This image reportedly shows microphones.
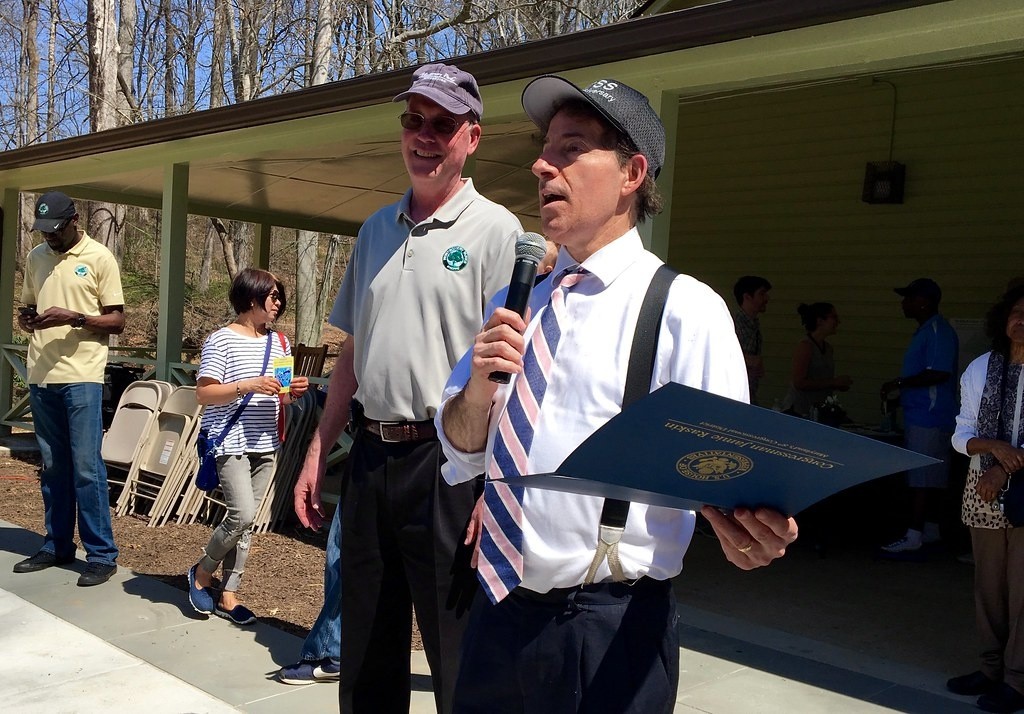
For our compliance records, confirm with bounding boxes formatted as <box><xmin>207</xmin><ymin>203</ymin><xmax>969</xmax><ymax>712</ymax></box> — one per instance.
<box><xmin>488</xmin><ymin>232</ymin><xmax>547</xmax><ymax>384</ymax></box>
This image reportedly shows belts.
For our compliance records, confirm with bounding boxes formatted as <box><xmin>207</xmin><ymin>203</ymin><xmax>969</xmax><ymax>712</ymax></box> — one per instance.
<box><xmin>511</xmin><ymin>584</ymin><xmax>646</xmax><ymax>605</ymax></box>
<box><xmin>364</xmin><ymin>417</ymin><xmax>419</xmax><ymax>442</ymax></box>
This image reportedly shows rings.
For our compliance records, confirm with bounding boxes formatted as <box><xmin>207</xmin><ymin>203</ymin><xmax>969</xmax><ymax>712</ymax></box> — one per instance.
<box><xmin>738</xmin><ymin>535</ymin><xmax>754</xmax><ymax>552</ymax></box>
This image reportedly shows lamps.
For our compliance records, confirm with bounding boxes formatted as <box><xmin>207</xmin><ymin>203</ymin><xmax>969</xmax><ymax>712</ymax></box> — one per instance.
<box><xmin>862</xmin><ymin>159</ymin><xmax>906</xmax><ymax>205</ymax></box>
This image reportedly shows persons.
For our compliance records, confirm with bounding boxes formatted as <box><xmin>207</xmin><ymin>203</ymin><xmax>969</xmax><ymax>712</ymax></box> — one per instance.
<box><xmin>781</xmin><ymin>302</ymin><xmax>853</xmax><ymax>415</ymax></box>
<box><xmin>296</xmin><ymin>63</ymin><xmax>524</xmax><ymax>714</ymax></box>
<box><xmin>880</xmin><ymin>278</ymin><xmax>959</xmax><ymax>568</ymax></box>
<box><xmin>13</xmin><ymin>189</ymin><xmax>126</xmax><ymax>584</ymax></box>
<box><xmin>946</xmin><ymin>284</ymin><xmax>1024</xmax><ymax>714</ymax></box>
<box><xmin>434</xmin><ymin>74</ymin><xmax>798</xmax><ymax>714</ymax></box>
<box><xmin>537</xmin><ymin>241</ymin><xmax>558</xmax><ymax>274</ymax></box>
<box><xmin>188</xmin><ymin>267</ymin><xmax>309</xmax><ymax>625</ymax></box>
<box><xmin>278</xmin><ymin>503</ymin><xmax>342</xmax><ymax>683</ymax></box>
<box><xmin>696</xmin><ymin>275</ymin><xmax>772</xmax><ymax>538</ymax></box>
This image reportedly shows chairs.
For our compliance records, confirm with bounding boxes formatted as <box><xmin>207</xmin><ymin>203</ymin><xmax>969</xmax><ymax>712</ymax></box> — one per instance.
<box><xmin>100</xmin><ymin>341</ymin><xmax>328</xmax><ymax>534</ymax></box>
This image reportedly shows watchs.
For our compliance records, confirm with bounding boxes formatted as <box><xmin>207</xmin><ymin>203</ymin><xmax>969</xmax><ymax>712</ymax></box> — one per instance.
<box><xmin>896</xmin><ymin>377</ymin><xmax>903</xmax><ymax>388</ymax></box>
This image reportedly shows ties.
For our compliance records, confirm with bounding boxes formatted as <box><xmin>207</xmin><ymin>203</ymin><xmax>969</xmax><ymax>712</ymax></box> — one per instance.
<box><xmin>477</xmin><ymin>268</ymin><xmax>591</xmax><ymax>605</ymax></box>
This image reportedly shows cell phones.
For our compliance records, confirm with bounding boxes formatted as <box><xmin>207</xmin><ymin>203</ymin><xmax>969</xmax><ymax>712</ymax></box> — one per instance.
<box><xmin>18</xmin><ymin>307</ymin><xmax>38</xmax><ymax>318</ymax></box>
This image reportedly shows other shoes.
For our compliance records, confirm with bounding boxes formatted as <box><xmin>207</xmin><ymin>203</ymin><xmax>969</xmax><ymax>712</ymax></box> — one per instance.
<box><xmin>187</xmin><ymin>563</ymin><xmax>213</xmax><ymax>614</ymax></box>
<box><xmin>976</xmin><ymin>680</ymin><xmax>1024</xmax><ymax>712</ymax></box>
<box><xmin>215</xmin><ymin>596</ymin><xmax>257</xmax><ymax>624</ymax></box>
<box><xmin>881</xmin><ymin>529</ymin><xmax>921</xmax><ymax>552</ymax></box>
<box><xmin>945</xmin><ymin>671</ymin><xmax>992</xmax><ymax>695</ymax></box>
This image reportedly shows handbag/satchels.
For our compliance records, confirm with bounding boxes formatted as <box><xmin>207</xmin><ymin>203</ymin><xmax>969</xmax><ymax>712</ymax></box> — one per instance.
<box><xmin>194</xmin><ymin>428</ymin><xmax>220</xmax><ymax>491</ymax></box>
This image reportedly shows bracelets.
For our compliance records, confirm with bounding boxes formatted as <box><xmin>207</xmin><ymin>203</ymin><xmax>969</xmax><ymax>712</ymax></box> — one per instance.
<box><xmin>237</xmin><ymin>384</ymin><xmax>241</xmax><ymax>397</ymax></box>
<box><xmin>78</xmin><ymin>313</ymin><xmax>85</xmax><ymax>327</ymax></box>
<box><xmin>289</xmin><ymin>391</ymin><xmax>303</xmax><ymax>401</ymax></box>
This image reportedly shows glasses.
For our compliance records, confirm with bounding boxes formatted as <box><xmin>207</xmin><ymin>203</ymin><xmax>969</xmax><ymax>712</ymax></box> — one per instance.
<box><xmin>268</xmin><ymin>290</ymin><xmax>284</xmax><ymax>302</ymax></box>
<box><xmin>398</xmin><ymin>111</ymin><xmax>469</xmax><ymax>134</ymax></box>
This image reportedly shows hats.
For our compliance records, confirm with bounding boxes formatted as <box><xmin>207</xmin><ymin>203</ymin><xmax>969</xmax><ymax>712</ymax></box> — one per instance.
<box><xmin>29</xmin><ymin>190</ymin><xmax>75</xmax><ymax>233</ymax></box>
<box><xmin>521</xmin><ymin>74</ymin><xmax>665</xmax><ymax>182</ymax></box>
<box><xmin>894</xmin><ymin>278</ymin><xmax>942</xmax><ymax>305</ymax></box>
<box><xmin>392</xmin><ymin>63</ymin><xmax>483</xmax><ymax>122</ymax></box>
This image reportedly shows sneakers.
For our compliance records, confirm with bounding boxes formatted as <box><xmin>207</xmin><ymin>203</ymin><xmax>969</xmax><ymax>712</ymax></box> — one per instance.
<box><xmin>277</xmin><ymin>657</ymin><xmax>340</xmax><ymax>684</ymax></box>
<box><xmin>77</xmin><ymin>562</ymin><xmax>117</xmax><ymax>585</ymax></box>
<box><xmin>14</xmin><ymin>551</ymin><xmax>71</xmax><ymax>572</ymax></box>
